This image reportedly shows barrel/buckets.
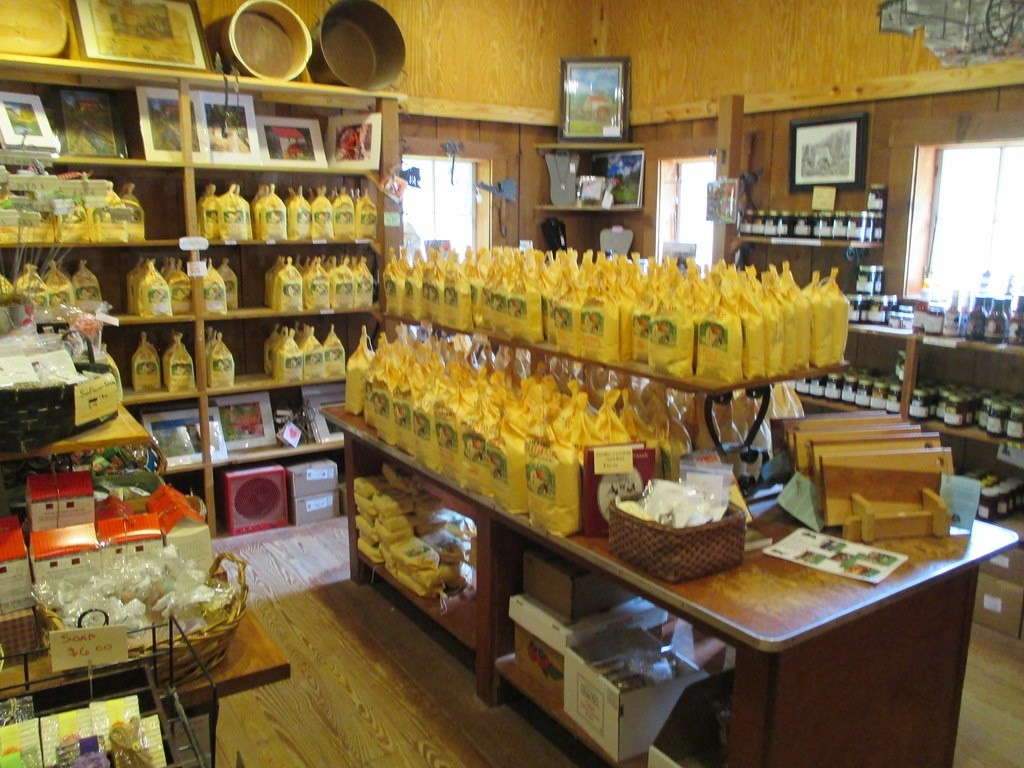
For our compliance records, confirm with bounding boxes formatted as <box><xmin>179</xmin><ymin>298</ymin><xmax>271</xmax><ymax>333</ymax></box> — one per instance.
<box><xmin>307</xmin><ymin>0</ymin><xmax>405</xmax><ymax>93</ymax></box>
<box><xmin>219</xmin><ymin>0</ymin><xmax>312</xmax><ymax>83</ymax></box>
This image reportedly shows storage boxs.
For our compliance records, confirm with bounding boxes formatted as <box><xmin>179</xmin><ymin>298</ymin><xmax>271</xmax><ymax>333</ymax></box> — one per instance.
<box><xmin>509</xmin><ymin>546</ymin><xmax>735</xmax><ymax>768</ymax></box>
<box><xmin>225</xmin><ymin>460</ymin><xmax>347</xmax><ymax>536</ymax></box>
<box><xmin>971</xmin><ymin>549</ymin><xmax>1024</xmax><ymax>639</ymax></box>
<box><xmin>0</xmin><ymin>471</ymin><xmax>211</xmax><ymax>657</ymax></box>
<box><xmin>0</xmin><ymin>149</ymin><xmax>107</xmax><ymax>226</ymax></box>
<box><xmin>0</xmin><ymin>695</ymin><xmax>168</xmax><ymax>768</ymax></box>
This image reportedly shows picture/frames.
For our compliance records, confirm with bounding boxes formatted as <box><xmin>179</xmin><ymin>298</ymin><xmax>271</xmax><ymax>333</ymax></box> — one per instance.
<box><xmin>0</xmin><ymin>85</ymin><xmax>383</xmax><ymax>171</ymax></box>
<box><xmin>788</xmin><ymin>110</ymin><xmax>869</xmax><ymax>192</ymax></box>
<box><xmin>558</xmin><ymin>55</ymin><xmax>631</xmax><ymax>144</ymax></box>
<box><xmin>140</xmin><ymin>383</ymin><xmax>345</xmax><ymax>468</ymax></box>
<box><xmin>70</xmin><ymin>0</ymin><xmax>215</xmax><ymax>72</ymax></box>
<box><xmin>591</xmin><ymin>150</ymin><xmax>645</xmax><ymax>209</ymax></box>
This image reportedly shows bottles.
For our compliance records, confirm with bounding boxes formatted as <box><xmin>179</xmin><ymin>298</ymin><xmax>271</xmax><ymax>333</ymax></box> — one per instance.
<box><xmin>941</xmin><ymin>290</ymin><xmax>1024</xmax><ymax>347</ymax></box>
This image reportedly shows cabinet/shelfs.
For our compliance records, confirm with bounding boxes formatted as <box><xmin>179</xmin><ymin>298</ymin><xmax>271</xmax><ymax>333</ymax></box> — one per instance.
<box><xmin>532</xmin><ymin>143</ymin><xmax>645</xmax><ymax>210</ymax></box>
<box><xmin>793</xmin><ymin>322</ymin><xmax>1024</xmax><ymax>548</ymax></box>
<box><xmin>0</xmin><ymin>404</ymin><xmax>291</xmax><ymax>713</ymax></box>
<box><xmin>0</xmin><ymin>53</ymin><xmax>409</xmax><ymax>538</ymax></box>
<box><xmin>318</xmin><ymin>313</ymin><xmax>1020</xmax><ymax>768</ymax></box>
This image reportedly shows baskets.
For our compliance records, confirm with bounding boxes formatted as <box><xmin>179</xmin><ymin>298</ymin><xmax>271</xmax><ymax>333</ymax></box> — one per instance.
<box><xmin>40</xmin><ymin>553</ymin><xmax>249</xmax><ymax>681</ymax></box>
<box><xmin>607</xmin><ymin>491</ymin><xmax>748</xmax><ymax>579</ymax></box>
